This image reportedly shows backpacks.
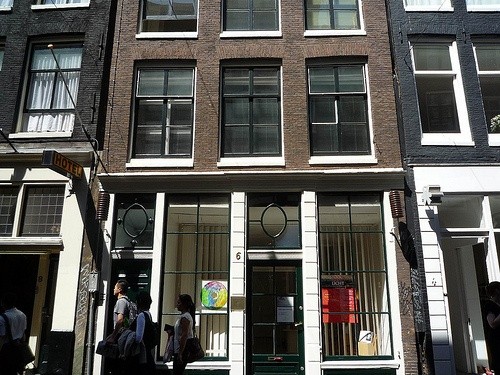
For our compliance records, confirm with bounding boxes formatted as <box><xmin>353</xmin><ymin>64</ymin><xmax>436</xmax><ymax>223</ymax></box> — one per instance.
<box><xmin>118</xmin><ymin>296</ymin><xmax>138</xmax><ymax>327</ymax></box>
<box><xmin>140</xmin><ymin>311</ymin><xmax>163</xmax><ymax>350</ymax></box>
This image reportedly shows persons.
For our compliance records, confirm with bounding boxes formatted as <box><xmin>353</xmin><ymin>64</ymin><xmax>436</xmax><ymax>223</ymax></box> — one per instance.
<box><xmin>102</xmin><ymin>279</ymin><xmax>133</xmax><ymax>375</ymax></box>
<box><xmin>163</xmin><ymin>294</ymin><xmax>195</xmax><ymax>375</ymax></box>
<box><xmin>482</xmin><ymin>281</ymin><xmax>500</xmax><ymax>375</ymax></box>
<box><xmin>1</xmin><ymin>293</ymin><xmax>34</xmax><ymax>375</ymax></box>
<box><xmin>126</xmin><ymin>288</ymin><xmax>156</xmax><ymax>375</ymax></box>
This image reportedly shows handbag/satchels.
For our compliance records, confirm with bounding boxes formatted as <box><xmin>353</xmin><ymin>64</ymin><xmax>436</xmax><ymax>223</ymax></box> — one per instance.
<box><xmin>96</xmin><ymin>340</ymin><xmax>108</xmax><ymax>356</ymax></box>
<box><xmin>2</xmin><ymin>339</ymin><xmax>35</xmax><ymax>374</ymax></box>
<box><xmin>178</xmin><ymin>336</ymin><xmax>204</xmax><ymax>363</ymax></box>
